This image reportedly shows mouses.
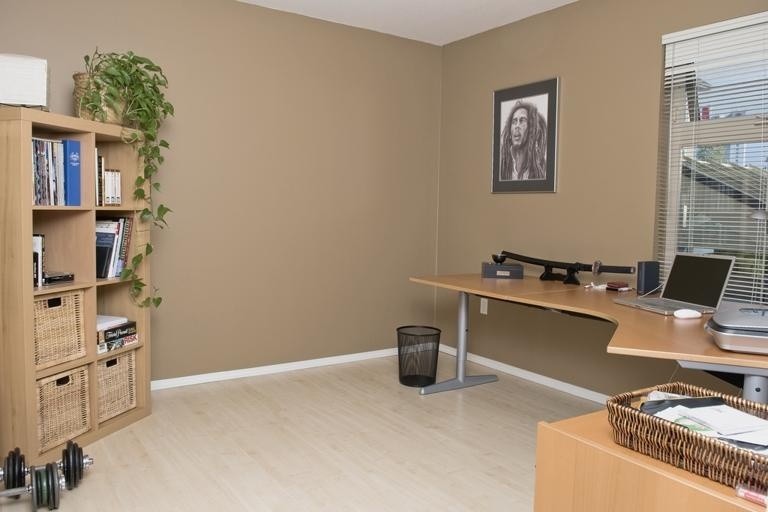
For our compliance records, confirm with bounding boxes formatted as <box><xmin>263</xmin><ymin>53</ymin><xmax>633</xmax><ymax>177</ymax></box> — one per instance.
<box><xmin>674</xmin><ymin>309</ymin><xmax>702</xmax><ymax>319</ymax></box>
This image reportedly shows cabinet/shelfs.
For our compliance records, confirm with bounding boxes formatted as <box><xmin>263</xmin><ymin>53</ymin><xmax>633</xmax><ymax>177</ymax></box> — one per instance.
<box><xmin>0</xmin><ymin>108</ymin><xmax>151</xmax><ymax>470</ymax></box>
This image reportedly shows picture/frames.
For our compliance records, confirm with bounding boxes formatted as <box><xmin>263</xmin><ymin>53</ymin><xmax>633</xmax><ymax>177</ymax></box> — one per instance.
<box><xmin>490</xmin><ymin>76</ymin><xmax>559</xmax><ymax>195</ymax></box>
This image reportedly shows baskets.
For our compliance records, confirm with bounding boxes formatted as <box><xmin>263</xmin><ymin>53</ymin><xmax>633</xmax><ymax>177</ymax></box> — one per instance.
<box><xmin>607</xmin><ymin>383</ymin><xmax>768</xmax><ymax>494</ymax></box>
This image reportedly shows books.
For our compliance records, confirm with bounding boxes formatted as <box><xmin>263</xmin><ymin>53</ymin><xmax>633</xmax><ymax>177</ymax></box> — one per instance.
<box><xmin>96</xmin><ymin>313</ymin><xmax>127</xmax><ymax>332</ymax></box>
<box><xmin>96</xmin><ymin>320</ymin><xmax>137</xmax><ymax>346</ymax></box>
<box><xmin>33</xmin><ymin>136</ymin><xmax>135</xmax><ymax>287</ymax></box>
<box><xmin>97</xmin><ymin>333</ymin><xmax>139</xmax><ymax>355</ymax></box>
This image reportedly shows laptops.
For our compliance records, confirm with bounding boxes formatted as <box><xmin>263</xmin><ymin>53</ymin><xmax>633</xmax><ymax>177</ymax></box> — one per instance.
<box><xmin>613</xmin><ymin>251</ymin><xmax>738</xmax><ymax>316</ymax></box>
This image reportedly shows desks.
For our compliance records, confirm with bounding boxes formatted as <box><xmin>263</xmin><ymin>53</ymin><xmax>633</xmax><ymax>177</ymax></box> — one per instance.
<box><xmin>409</xmin><ymin>272</ymin><xmax>768</xmax><ymax>405</ymax></box>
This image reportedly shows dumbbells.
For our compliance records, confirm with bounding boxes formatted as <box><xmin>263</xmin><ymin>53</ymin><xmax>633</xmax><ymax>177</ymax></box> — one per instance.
<box><xmin>0</xmin><ymin>440</ymin><xmax>94</xmax><ymax>512</ymax></box>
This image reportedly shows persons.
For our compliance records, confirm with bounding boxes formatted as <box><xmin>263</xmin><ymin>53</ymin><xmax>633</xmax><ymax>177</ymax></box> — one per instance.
<box><xmin>497</xmin><ymin>101</ymin><xmax>547</xmax><ymax>179</ymax></box>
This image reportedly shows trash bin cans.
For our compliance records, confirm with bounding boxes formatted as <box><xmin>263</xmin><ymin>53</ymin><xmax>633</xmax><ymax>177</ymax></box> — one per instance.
<box><xmin>395</xmin><ymin>325</ymin><xmax>442</xmax><ymax>401</ymax></box>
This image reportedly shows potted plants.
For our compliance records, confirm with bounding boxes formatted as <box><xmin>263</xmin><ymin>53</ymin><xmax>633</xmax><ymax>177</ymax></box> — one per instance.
<box><xmin>74</xmin><ymin>51</ymin><xmax>172</xmax><ymax>311</ymax></box>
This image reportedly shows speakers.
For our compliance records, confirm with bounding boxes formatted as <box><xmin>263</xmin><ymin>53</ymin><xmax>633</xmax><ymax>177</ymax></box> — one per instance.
<box><xmin>637</xmin><ymin>261</ymin><xmax>660</xmax><ymax>296</ymax></box>
